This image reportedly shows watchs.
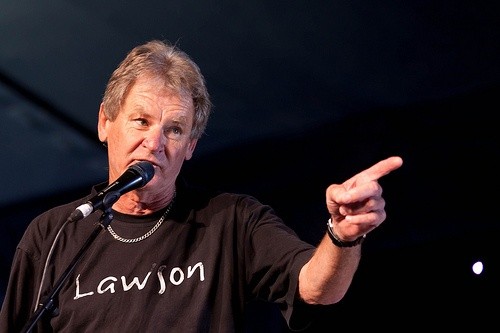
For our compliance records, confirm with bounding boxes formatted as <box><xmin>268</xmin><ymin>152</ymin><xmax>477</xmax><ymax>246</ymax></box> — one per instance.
<box><xmin>318</xmin><ymin>218</ymin><xmax>368</xmax><ymax>247</ymax></box>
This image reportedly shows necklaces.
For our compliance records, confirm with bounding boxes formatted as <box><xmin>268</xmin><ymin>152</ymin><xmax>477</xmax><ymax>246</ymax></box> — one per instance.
<box><xmin>92</xmin><ymin>190</ymin><xmax>179</xmax><ymax>244</ymax></box>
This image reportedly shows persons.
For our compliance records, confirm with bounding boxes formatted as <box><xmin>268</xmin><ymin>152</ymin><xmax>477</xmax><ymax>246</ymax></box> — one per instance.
<box><xmin>3</xmin><ymin>41</ymin><xmax>405</xmax><ymax>333</ymax></box>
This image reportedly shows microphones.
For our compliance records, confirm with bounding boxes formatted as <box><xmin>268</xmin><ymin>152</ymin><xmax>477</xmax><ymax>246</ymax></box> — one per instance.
<box><xmin>66</xmin><ymin>161</ymin><xmax>156</xmax><ymax>222</ymax></box>
